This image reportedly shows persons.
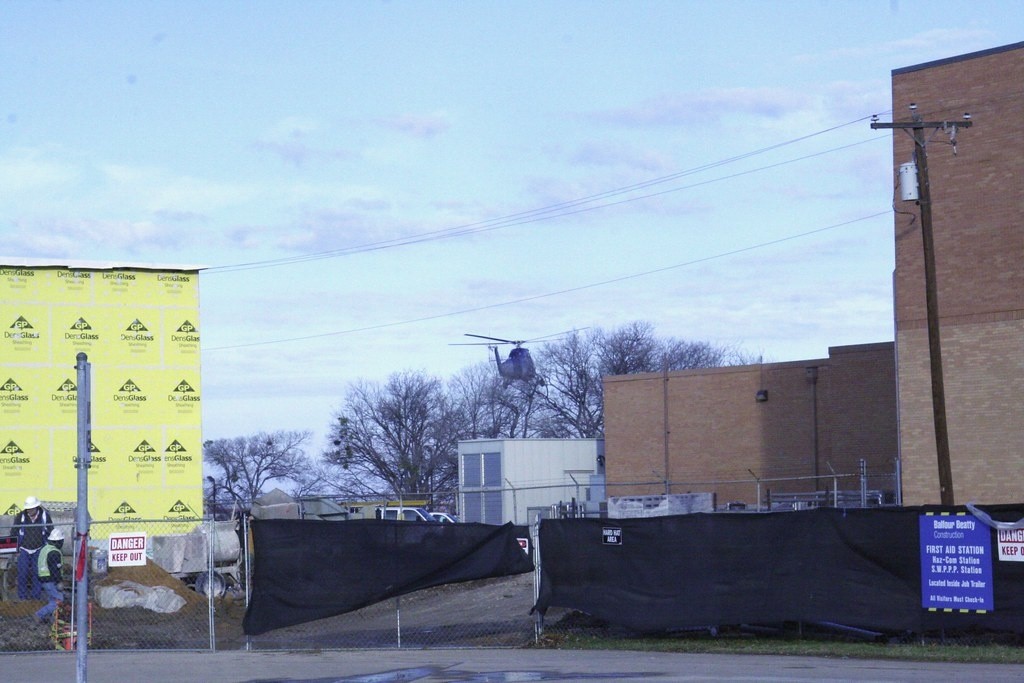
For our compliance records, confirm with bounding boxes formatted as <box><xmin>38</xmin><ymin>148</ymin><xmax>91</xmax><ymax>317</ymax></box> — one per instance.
<box><xmin>9</xmin><ymin>496</ymin><xmax>54</xmax><ymax>603</ymax></box>
<box><xmin>32</xmin><ymin>529</ymin><xmax>66</xmax><ymax>625</ymax></box>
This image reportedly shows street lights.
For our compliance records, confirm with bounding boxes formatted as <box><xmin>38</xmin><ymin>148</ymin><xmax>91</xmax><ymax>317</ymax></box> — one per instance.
<box><xmin>206</xmin><ymin>476</ymin><xmax>216</xmax><ymax>521</ymax></box>
<box><xmin>424</xmin><ymin>445</ymin><xmax>434</xmax><ymax>511</ymax></box>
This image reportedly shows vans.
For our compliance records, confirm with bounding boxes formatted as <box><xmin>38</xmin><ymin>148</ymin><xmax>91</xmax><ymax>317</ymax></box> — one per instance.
<box><xmin>375</xmin><ymin>507</ymin><xmax>438</xmax><ymax>523</ymax></box>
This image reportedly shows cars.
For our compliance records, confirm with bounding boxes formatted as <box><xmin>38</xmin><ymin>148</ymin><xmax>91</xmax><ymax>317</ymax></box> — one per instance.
<box><xmin>427</xmin><ymin>511</ymin><xmax>461</xmax><ymax>524</ymax></box>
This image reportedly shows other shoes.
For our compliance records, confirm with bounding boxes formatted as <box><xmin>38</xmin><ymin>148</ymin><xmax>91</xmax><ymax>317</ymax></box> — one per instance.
<box><xmin>31</xmin><ymin>613</ymin><xmax>42</xmax><ymax>627</ymax></box>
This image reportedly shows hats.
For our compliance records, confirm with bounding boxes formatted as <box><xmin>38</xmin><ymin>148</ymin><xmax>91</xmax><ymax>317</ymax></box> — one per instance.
<box><xmin>24</xmin><ymin>496</ymin><xmax>41</xmax><ymax>510</ymax></box>
<box><xmin>47</xmin><ymin>528</ymin><xmax>64</xmax><ymax>540</ymax></box>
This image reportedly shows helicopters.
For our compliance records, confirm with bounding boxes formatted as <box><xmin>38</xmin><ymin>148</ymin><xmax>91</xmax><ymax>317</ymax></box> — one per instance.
<box><xmin>446</xmin><ymin>325</ymin><xmax>592</xmax><ymax>390</ymax></box>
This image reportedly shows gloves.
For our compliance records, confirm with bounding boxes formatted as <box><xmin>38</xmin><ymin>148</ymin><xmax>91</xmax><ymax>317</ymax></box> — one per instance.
<box><xmin>57</xmin><ymin>582</ymin><xmax>66</xmax><ymax>591</ymax></box>
